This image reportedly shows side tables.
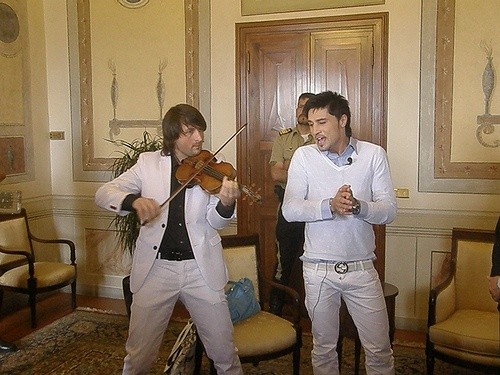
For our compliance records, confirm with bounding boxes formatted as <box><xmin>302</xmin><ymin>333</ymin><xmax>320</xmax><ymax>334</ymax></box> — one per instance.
<box><xmin>336</xmin><ymin>281</ymin><xmax>399</xmax><ymax>375</ymax></box>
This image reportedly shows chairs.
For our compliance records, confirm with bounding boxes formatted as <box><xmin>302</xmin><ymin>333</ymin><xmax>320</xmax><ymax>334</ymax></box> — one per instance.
<box><xmin>0</xmin><ymin>208</ymin><xmax>78</xmax><ymax>329</ymax></box>
<box><xmin>193</xmin><ymin>231</ymin><xmax>303</xmax><ymax>375</ymax></box>
<box><xmin>425</xmin><ymin>228</ymin><xmax>500</xmax><ymax>375</ymax></box>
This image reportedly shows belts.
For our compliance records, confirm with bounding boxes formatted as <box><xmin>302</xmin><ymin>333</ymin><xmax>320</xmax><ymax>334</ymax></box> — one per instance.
<box><xmin>305</xmin><ymin>260</ymin><xmax>375</xmax><ymax>274</ymax></box>
<box><xmin>156</xmin><ymin>251</ymin><xmax>195</xmax><ymax>261</ymax></box>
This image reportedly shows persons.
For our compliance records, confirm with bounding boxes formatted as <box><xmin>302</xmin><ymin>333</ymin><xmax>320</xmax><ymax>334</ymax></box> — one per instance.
<box><xmin>269</xmin><ymin>92</ymin><xmax>316</xmax><ymax>315</ymax></box>
<box><xmin>94</xmin><ymin>103</ymin><xmax>243</xmax><ymax>375</ymax></box>
<box><xmin>281</xmin><ymin>90</ymin><xmax>398</xmax><ymax>375</ymax></box>
<box><xmin>487</xmin><ymin>216</ymin><xmax>500</xmax><ymax>313</ymax></box>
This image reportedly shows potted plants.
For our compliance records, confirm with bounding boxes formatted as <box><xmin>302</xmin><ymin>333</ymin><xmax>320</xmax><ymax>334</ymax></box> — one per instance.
<box><xmin>103</xmin><ymin>131</ymin><xmax>163</xmax><ymax>321</ymax></box>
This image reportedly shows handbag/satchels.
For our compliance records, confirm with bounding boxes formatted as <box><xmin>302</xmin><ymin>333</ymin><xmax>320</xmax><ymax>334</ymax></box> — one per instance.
<box><xmin>225</xmin><ymin>278</ymin><xmax>262</xmax><ymax>322</ymax></box>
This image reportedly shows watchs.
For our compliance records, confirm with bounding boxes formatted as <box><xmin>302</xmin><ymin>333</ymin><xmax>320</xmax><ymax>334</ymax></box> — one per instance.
<box><xmin>351</xmin><ymin>198</ymin><xmax>360</xmax><ymax>215</ymax></box>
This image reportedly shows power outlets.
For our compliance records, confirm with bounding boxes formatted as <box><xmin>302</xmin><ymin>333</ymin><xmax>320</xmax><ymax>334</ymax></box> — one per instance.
<box><xmin>49</xmin><ymin>132</ymin><xmax>64</xmax><ymax>141</ymax></box>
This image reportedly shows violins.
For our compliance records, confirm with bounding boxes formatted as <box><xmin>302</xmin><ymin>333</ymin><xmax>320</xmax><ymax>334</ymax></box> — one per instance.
<box><xmin>175</xmin><ymin>150</ymin><xmax>263</xmax><ymax>207</ymax></box>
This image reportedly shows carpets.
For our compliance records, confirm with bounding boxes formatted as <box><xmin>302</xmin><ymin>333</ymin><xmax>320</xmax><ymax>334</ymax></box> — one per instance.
<box><xmin>0</xmin><ymin>308</ymin><xmax>483</xmax><ymax>375</ymax></box>
